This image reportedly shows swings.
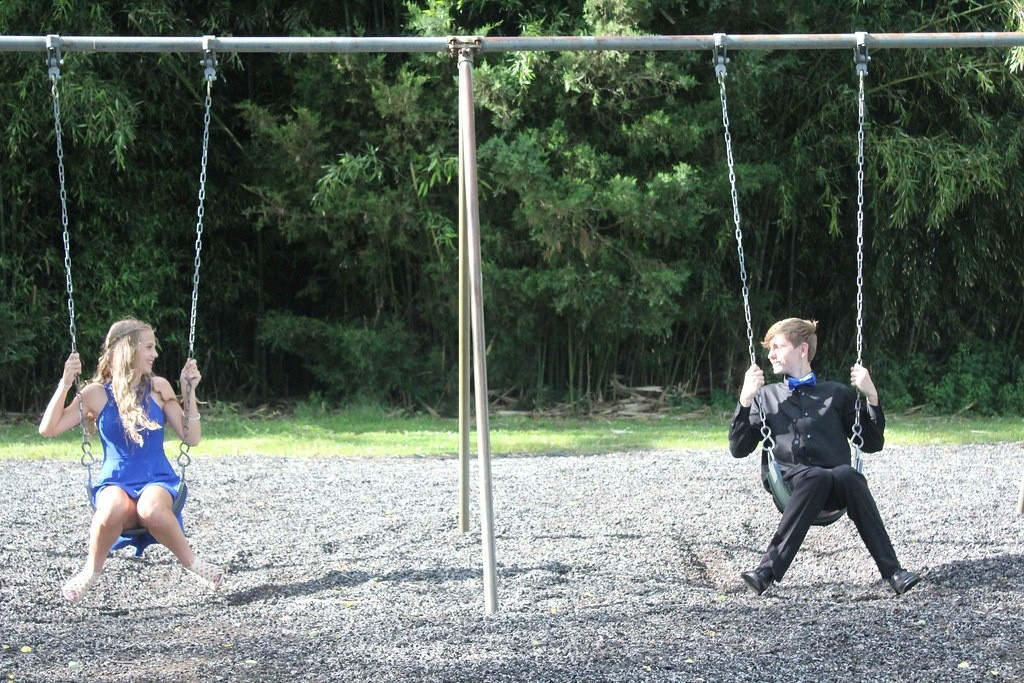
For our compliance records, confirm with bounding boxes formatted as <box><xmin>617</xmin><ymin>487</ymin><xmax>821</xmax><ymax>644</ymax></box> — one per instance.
<box><xmin>40</xmin><ymin>54</ymin><xmax>222</xmax><ymax>545</ymax></box>
<box><xmin>707</xmin><ymin>52</ymin><xmax>880</xmax><ymax>531</ymax></box>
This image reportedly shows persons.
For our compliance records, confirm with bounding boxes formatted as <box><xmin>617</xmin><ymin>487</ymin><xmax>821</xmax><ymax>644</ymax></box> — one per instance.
<box><xmin>37</xmin><ymin>320</ymin><xmax>225</xmax><ymax>600</ymax></box>
<box><xmin>728</xmin><ymin>318</ymin><xmax>922</xmax><ymax>596</ymax></box>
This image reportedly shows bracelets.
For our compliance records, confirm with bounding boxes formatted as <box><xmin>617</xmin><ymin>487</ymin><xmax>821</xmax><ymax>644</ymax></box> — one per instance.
<box><xmin>183</xmin><ymin>412</ymin><xmax>201</xmax><ymax>421</ymax></box>
<box><xmin>60</xmin><ymin>379</ymin><xmax>72</xmax><ymax>390</ymax></box>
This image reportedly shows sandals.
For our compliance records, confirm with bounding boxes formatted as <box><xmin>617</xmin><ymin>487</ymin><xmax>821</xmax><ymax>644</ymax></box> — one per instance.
<box><xmin>187</xmin><ymin>554</ymin><xmax>226</xmax><ymax>591</ymax></box>
<box><xmin>60</xmin><ymin>568</ymin><xmax>103</xmax><ymax>604</ymax></box>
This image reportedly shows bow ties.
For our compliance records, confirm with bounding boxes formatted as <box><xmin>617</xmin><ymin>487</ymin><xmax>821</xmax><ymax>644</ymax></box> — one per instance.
<box><xmin>788</xmin><ymin>372</ymin><xmax>816</xmax><ymax>391</ymax></box>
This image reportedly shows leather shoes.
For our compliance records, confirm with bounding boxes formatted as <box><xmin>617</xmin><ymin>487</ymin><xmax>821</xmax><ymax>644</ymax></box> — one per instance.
<box><xmin>886</xmin><ymin>567</ymin><xmax>923</xmax><ymax>596</ymax></box>
<box><xmin>740</xmin><ymin>563</ymin><xmax>777</xmax><ymax>595</ymax></box>
